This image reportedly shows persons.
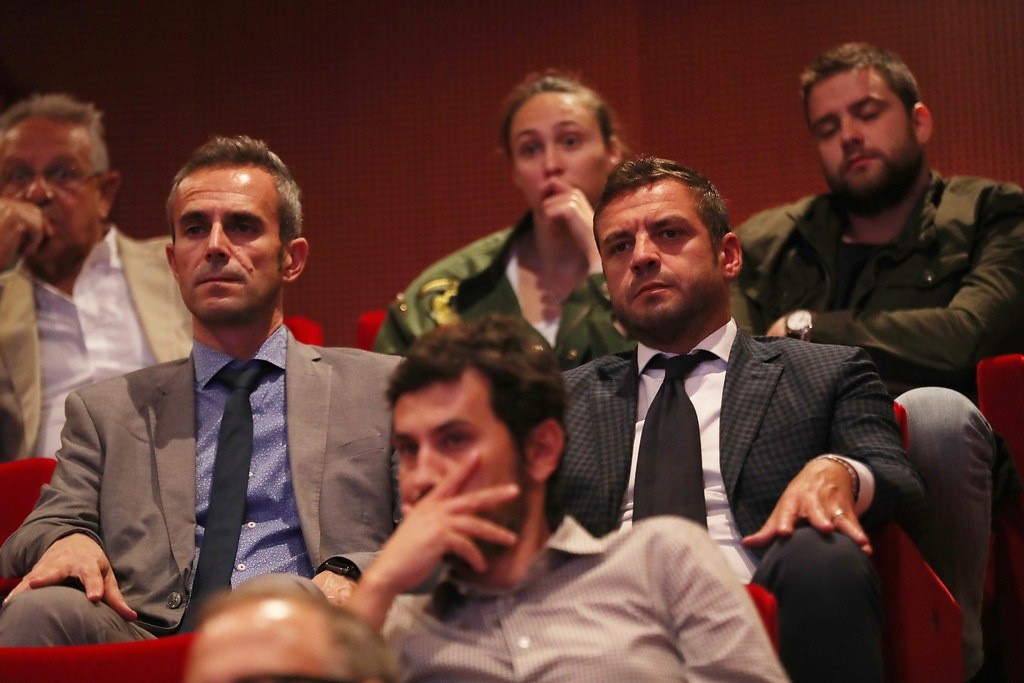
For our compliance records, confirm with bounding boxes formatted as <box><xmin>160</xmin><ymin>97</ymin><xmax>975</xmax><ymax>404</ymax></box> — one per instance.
<box><xmin>551</xmin><ymin>157</ymin><xmax>910</xmax><ymax>683</ymax></box>
<box><xmin>0</xmin><ymin>94</ymin><xmax>191</xmax><ymax>466</ymax></box>
<box><xmin>372</xmin><ymin>70</ymin><xmax>639</xmax><ymax>371</ymax></box>
<box><xmin>731</xmin><ymin>43</ymin><xmax>1024</xmax><ymax>683</ymax></box>
<box><xmin>0</xmin><ymin>136</ymin><xmax>407</xmax><ymax>646</ymax></box>
<box><xmin>187</xmin><ymin>313</ymin><xmax>793</xmax><ymax>683</ymax></box>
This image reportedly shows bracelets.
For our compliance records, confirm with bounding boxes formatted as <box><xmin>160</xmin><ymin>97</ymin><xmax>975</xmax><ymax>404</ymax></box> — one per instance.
<box><xmin>806</xmin><ymin>456</ymin><xmax>857</xmax><ymax>502</ymax></box>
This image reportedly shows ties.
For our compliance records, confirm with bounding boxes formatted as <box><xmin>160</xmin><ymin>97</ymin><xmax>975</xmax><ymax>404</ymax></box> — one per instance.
<box><xmin>176</xmin><ymin>359</ymin><xmax>267</xmax><ymax>636</ymax></box>
<box><xmin>632</xmin><ymin>349</ymin><xmax>718</xmax><ymax>533</ymax></box>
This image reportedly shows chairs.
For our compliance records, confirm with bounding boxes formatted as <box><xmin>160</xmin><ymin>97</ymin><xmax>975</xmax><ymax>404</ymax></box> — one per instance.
<box><xmin>0</xmin><ymin>310</ymin><xmax>1024</xmax><ymax>683</ymax></box>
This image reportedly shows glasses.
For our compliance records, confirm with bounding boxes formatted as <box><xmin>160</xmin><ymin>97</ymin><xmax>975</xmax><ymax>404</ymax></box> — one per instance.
<box><xmin>0</xmin><ymin>164</ymin><xmax>106</xmax><ymax>198</ymax></box>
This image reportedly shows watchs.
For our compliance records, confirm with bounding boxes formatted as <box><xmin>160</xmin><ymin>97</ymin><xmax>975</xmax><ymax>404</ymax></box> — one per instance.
<box><xmin>784</xmin><ymin>311</ymin><xmax>815</xmax><ymax>341</ymax></box>
<box><xmin>316</xmin><ymin>557</ymin><xmax>362</xmax><ymax>584</ymax></box>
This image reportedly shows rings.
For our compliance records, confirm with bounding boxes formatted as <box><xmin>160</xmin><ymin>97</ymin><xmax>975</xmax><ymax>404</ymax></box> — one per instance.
<box><xmin>831</xmin><ymin>509</ymin><xmax>844</xmax><ymax>518</ymax></box>
